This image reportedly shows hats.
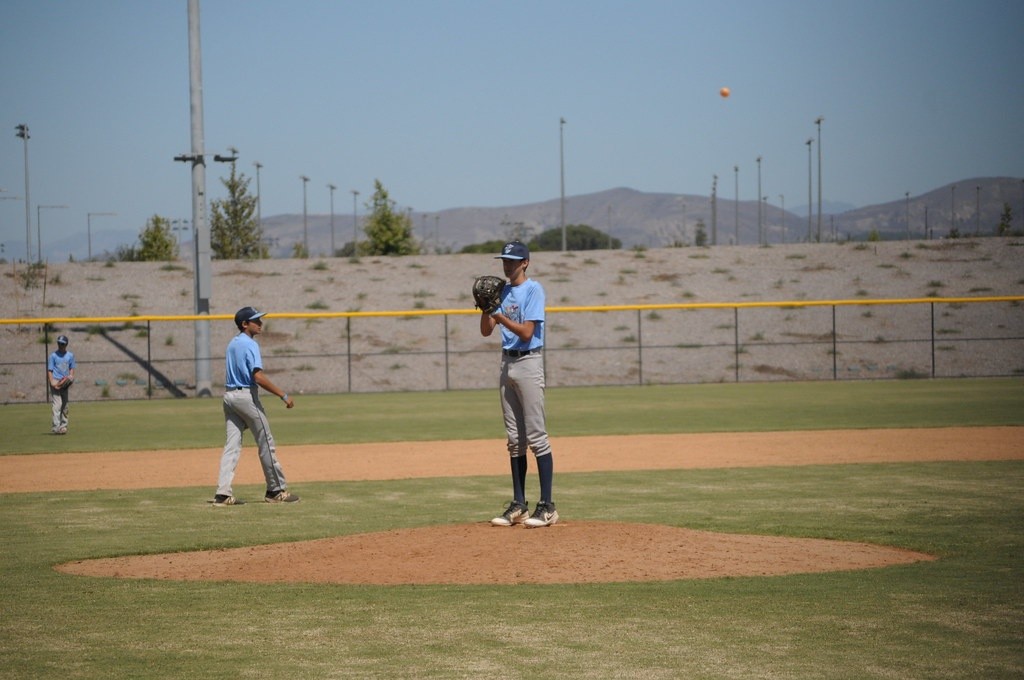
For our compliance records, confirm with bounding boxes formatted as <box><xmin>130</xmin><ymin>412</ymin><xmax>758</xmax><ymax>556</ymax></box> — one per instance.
<box><xmin>56</xmin><ymin>335</ymin><xmax>68</xmax><ymax>345</ymax></box>
<box><xmin>234</xmin><ymin>306</ymin><xmax>267</xmax><ymax>326</ymax></box>
<box><xmin>493</xmin><ymin>240</ymin><xmax>529</xmax><ymax>261</ymax></box>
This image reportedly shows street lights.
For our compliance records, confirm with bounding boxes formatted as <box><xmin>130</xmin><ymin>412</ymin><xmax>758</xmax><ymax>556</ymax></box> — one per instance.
<box><xmin>814</xmin><ymin>115</ymin><xmax>825</xmax><ymax>242</ymax></box>
<box><xmin>735</xmin><ymin>164</ymin><xmax>739</xmax><ymax>245</ymax></box>
<box><xmin>906</xmin><ymin>191</ymin><xmax>912</xmax><ymax>240</ymax></box>
<box><xmin>328</xmin><ymin>183</ymin><xmax>339</xmax><ymax>257</ymax></box>
<box><xmin>805</xmin><ymin>137</ymin><xmax>815</xmax><ymax>242</ymax></box>
<box><xmin>351</xmin><ymin>189</ymin><xmax>360</xmax><ymax>256</ymax></box>
<box><xmin>14</xmin><ymin>122</ymin><xmax>33</xmax><ymax>265</ymax></box>
<box><xmin>755</xmin><ymin>156</ymin><xmax>764</xmax><ymax>244</ymax></box>
<box><xmin>256</xmin><ymin>163</ymin><xmax>264</xmax><ymax>259</ymax></box>
<box><xmin>299</xmin><ymin>174</ymin><xmax>311</xmax><ymax>259</ymax></box>
<box><xmin>560</xmin><ymin>115</ymin><xmax>568</xmax><ymax>251</ymax></box>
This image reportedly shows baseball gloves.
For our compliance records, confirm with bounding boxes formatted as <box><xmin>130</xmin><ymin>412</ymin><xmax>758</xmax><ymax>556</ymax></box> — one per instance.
<box><xmin>58</xmin><ymin>375</ymin><xmax>74</xmax><ymax>390</ymax></box>
<box><xmin>472</xmin><ymin>275</ymin><xmax>507</xmax><ymax>315</ymax></box>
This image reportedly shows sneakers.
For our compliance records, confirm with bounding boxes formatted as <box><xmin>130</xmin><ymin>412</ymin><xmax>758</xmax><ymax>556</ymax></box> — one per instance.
<box><xmin>265</xmin><ymin>489</ymin><xmax>301</xmax><ymax>505</ymax></box>
<box><xmin>212</xmin><ymin>494</ymin><xmax>246</xmax><ymax>507</ymax></box>
<box><xmin>524</xmin><ymin>501</ymin><xmax>558</xmax><ymax>527</ymax></box>
<box><xmin>490</xmin><ymin>500</ymin><xmax>530</xmax><ymax>527</ymax></box>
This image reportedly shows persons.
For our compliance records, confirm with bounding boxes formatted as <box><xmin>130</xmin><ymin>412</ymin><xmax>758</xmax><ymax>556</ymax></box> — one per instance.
<box><xmin>214</xmin><ymin>307</ymin><xmax>301</xmax><ymax>507</ymax></box>
<box><xmin>48</xmin><ymin>335</ymin><xmax>75</xmax><ymax>434</ymax></box>
<box><xmin>472</xmin><ymin>240</ymin><xmax>559</xmax><ymax>528</ymax></box>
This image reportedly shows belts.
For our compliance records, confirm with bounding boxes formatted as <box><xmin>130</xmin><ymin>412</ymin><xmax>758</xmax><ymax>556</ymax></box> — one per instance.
<box><xmin>502</xmin><ymin>348</ymin><xmax>530</xmax><ymax>357</ymax></box>
<box><xmin>237</xmin><ymin>386</ymin><xmax>242</xmax><ymax>390</ymax></box>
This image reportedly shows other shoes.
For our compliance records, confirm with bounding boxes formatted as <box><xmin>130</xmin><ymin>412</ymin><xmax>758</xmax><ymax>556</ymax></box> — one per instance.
<box><xmin>60</xmin><ymin>428</ymin><xmax>67</xmax><ymax>433</ymax></box>
<box><xmin>50</xmin><ymin>426</ymin><xmax>58</xmax><ymax>433</ymax></box>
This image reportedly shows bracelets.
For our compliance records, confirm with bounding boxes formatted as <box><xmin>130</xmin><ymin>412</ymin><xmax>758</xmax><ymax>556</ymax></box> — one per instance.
<box><xmin>281</xmin><ymin>393</ymin><xmax>288</xmax><ymax>400</ymax></box>
